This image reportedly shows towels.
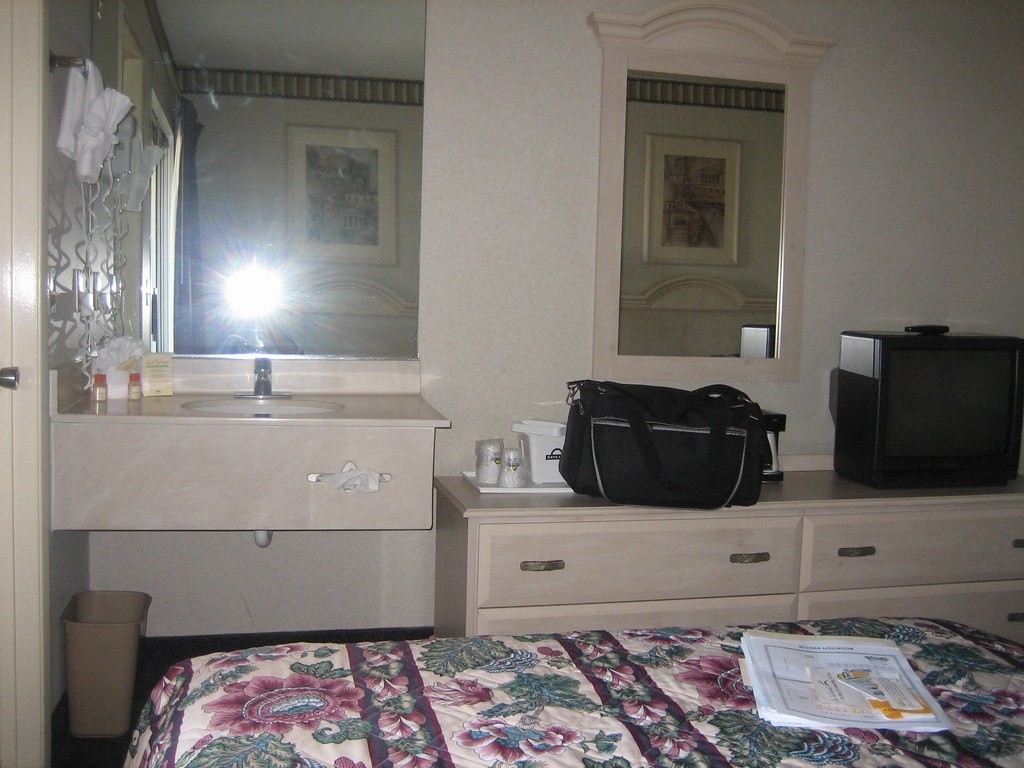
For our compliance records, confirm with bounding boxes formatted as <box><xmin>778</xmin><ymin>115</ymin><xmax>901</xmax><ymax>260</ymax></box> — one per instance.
<box><xmin>55</xmin><ymin>58</ymin><xmax>135</xmax><ymax>184</ymax></box>
<box><xmin>112</xmin><ymin>137</ymin><xmax>165</xmax><ymax>213</ymax></box>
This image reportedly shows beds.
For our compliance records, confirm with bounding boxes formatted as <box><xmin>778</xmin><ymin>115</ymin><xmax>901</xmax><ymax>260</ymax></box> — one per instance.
<box><xmin>121</xmin><ymin>615</ymin><xmax>1024</xmax><ymax>768</ymax></box>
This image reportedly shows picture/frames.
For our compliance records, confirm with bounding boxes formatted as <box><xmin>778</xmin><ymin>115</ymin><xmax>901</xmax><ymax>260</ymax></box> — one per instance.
<box><xmin>643</xmin><ymin>132</ymin><xmax>742</xmax><ymax>264</ymax></box>
<box><xmin>285</xmin><ymin>123</ymin><xmax>398</xmax><ymax>267</ymax></box>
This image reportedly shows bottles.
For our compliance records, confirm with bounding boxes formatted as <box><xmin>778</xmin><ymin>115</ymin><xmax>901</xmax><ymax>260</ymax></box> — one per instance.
<box><xmin>93</xmin><ymin>374</ymin><xmax>107</xmax><ymax>401</ymax></box>
<box><xmin>128</xmin><ymin>373</ymin><xmax>141</xmax><ymax>401</ymax></box>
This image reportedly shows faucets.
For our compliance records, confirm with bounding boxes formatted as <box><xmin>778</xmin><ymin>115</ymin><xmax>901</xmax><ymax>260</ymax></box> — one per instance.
<box><xmin>233</xmin><ymin>357</ymin><xmax>292</xmax><ymax>399</ymax></box>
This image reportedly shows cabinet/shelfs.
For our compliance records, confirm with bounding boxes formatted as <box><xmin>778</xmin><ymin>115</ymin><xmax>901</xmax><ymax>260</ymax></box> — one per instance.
<box><xmin>434</xmin><ymin>470</ymin><xmax>1024</xmax><ymax>651</ymax></box>
<box><xmin>50</xmin><ymin>422</ymin><xmax>435</xmax><ymax>530</ymax></box>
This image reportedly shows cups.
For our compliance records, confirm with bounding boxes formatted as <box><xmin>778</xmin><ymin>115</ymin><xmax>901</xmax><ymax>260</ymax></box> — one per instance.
<box><xmin>476</xmin><ymin>442</ymin><xmax>501</xmax><ymax>484</ymax></box>
<box><xmin>498</xmin><ymin>447</ymin><xmax>526</xmax><ymax>488</ymax></box>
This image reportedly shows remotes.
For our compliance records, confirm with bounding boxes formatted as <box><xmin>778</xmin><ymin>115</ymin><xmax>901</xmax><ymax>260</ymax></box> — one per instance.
<box><xmin>904</xmin><ymin>325</ymin><xmax>949</xmax><ymax>334</ymax></box>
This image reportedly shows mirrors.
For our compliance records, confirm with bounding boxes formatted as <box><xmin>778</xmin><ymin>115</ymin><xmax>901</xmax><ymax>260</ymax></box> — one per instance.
<box><xmin>88</xmin><ymin>0</ymin><xmax>429</xmax><ymax>360</ymax></box>
<box><xmin>587</xmin><ymin>0</ymin><xmax>838</xmax><ymax>386</ymax></box>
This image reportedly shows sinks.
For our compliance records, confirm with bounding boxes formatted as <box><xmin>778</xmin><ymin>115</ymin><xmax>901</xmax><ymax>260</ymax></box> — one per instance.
<box><xmin>180</xmin><ymin>397</ymin><xmax>344</xmax><ymax>415</ymax></box>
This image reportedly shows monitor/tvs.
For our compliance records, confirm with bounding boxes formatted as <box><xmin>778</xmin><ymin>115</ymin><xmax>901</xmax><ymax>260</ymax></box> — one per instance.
<box><xmin>832</xmin><ymin>329</ymin><xmax>1024</xmax><ymax>488</ymax></box>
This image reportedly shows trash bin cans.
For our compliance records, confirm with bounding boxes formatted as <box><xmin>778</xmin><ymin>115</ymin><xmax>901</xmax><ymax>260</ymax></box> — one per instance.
<box><xmin>61</xmin><ymin>588</ymin><xmax>151</xmax><ymax>739</ymax></box>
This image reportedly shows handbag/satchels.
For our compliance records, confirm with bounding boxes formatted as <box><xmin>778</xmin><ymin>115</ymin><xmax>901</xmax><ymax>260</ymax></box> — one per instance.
<box><xmin>559</xmin><ymin>380</ymin><xmax>786</xmax><ymax>510</ymax></box>
